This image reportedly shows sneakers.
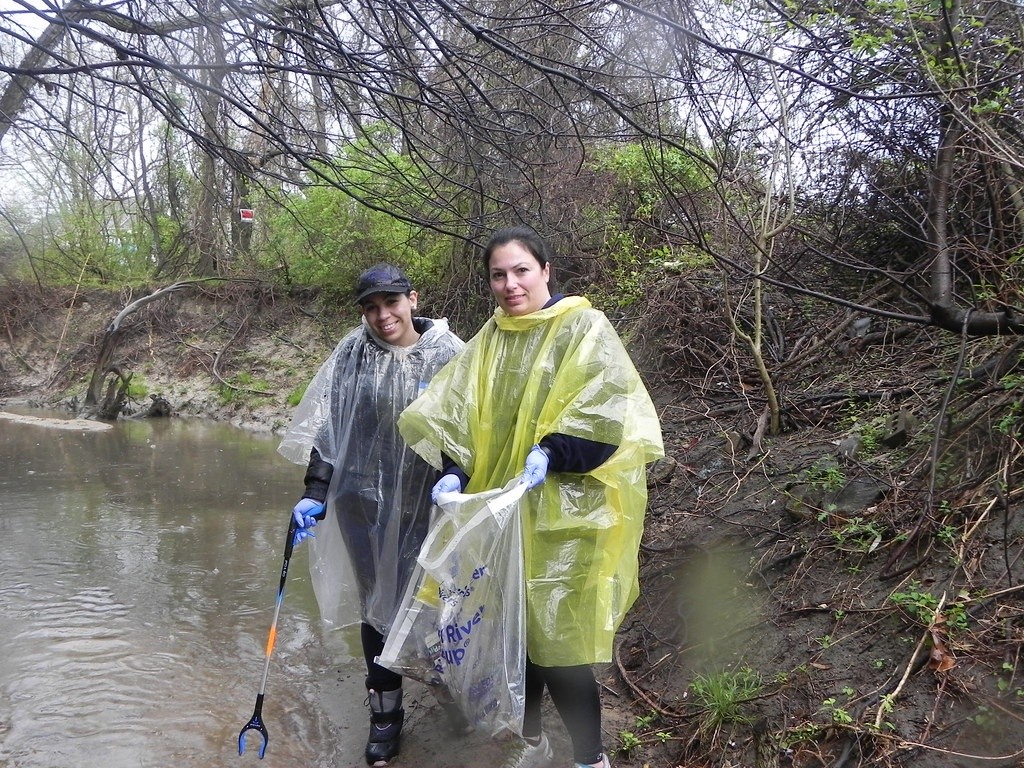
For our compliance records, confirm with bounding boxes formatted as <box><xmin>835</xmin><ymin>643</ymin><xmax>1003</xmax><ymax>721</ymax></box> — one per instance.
<box><xmin>501</xmin><ymin>733</ymin><xmax>554</xmax><ymax>768</ymax></box>
<box><xmin>572</xmin><ymin>753</ymin><xmax>612</xmax><ymax>768</ymax></box>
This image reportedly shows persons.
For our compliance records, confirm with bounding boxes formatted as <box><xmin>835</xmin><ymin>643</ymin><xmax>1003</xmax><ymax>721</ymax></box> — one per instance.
<box><xmin>431</xmin><ymin>226</ymin><xmax>629</xmax><ymax>768</ymax></box>
<box><xmin>292</xmin><ymin>264</ymin><xmax>476</xmax><ymax>767</ymax></box>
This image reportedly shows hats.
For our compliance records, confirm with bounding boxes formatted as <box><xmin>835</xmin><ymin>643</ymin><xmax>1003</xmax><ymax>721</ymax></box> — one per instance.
<box><xmin>354</xmin><ymin>264</ymin><xmax>412</xmax><ymax>307</ymax></box>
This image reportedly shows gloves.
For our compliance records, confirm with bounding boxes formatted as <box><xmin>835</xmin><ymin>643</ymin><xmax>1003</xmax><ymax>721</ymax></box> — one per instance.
<box><xmin>522</xmin><ymin>444</ymin><xmax>549</xmax><ymax>491</ymax></box>
<box><xmin>431</xmin><ymin>474</ymin><xmax>461</xmax><ymax>508</ymax></box>
<box><xmin>292</xmin><ymin>498</ymin><xmax>323</xmax><ymax>544</ymax></box>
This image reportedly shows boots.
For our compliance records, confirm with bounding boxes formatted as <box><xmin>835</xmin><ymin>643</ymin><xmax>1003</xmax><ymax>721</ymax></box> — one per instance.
<box><xmin>365</xmin><ymin>677</ymin><xmax>404</xmax><ymax>766</ymax></box>
<box><xmin>428</xmin><ymin>684</ymin><xmax>476</xmax><ymax>735</ymax></box>
